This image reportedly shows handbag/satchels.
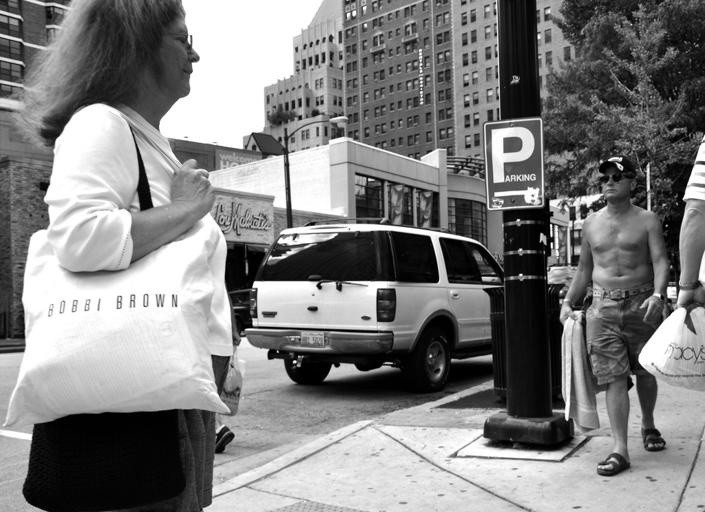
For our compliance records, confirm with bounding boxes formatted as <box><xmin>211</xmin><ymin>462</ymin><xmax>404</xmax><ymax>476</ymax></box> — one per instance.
<box><xmin>20</xmin><ymin>405</ymin><xmax>190</xmax><ymax>511</ymax></box>
<box><xmin>1</xmin><ymin>208</ymin><xmax>224</xmax><ymax>430</ymax></box>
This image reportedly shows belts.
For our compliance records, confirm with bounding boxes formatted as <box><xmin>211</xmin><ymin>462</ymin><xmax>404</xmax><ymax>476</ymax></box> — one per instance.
<box><xmin>592</xmin><ymin>281</ymin><xmax>655</xmax><ymax>302</ymax></box>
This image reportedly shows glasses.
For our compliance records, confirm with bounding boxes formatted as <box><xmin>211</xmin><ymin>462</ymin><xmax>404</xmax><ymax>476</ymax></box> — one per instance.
<box><xmin>600</xmin><ymin>172</ymin><xmax>635</xmax><ymax>183</ymax></box>
<box><xmin>157</xmin><ymin>33</ymin><xmax>193</xmax><ymax>56</ymax></box>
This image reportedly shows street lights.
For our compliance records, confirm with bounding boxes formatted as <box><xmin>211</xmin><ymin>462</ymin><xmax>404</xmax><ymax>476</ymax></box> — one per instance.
<box><xmin>284</xmin><ymin>116</ymin><xmax>349</xmax><ymax>228</ymax></box>
<box><xmin>560</xmin><ymin>202</ymin><xmax>574</xmax><ymax>266</ymax></box>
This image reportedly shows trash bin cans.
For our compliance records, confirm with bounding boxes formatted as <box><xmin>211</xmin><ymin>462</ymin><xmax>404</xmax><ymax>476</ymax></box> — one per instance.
<box><xmin>482</xmin><ymin>283</ymin><xmax>565</xmax><ymax>404</ymax></box>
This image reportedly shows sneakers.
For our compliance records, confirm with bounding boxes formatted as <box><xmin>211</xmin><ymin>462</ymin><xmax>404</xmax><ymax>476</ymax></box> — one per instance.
<box><xmin>212</xmin><ymin>424</ymin><xmax>235</xmax><ymax>454</ymax></box>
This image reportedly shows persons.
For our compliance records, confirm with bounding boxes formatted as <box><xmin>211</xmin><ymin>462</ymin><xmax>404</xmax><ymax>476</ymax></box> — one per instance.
<box><xmin>558</xmin><ymin>156</ymin><xmax>672</xmax><ymax>476</ymax></box>
<box><xmin>206</xmin><ymin>222</ymin><xmax>236</xmax><ymax>455</ymax></box>
<box><xmin>674</xmin><ymin>130</ymin><xmax>705</xmax><ymax>322</ymax></box>
<box><xmin>2</xmin><ymin>1</ymin><xmax>236</xmax><ymax>512</ymax></box>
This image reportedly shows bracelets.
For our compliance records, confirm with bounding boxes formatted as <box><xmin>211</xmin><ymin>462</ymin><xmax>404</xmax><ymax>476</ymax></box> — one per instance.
<box><xmin>678</xmin><ymin>279</ymin><xmax>702</xmax><ymax>290</ymax></box>
<box><xmin>652</xmin><ymin>292</ymin><xmax>666</xmax><ymax>302</ymax></box>
<box><xmin>562</xmin><ymin>298</ymin><xmax>572</xmax><ymax>303</ymax></box>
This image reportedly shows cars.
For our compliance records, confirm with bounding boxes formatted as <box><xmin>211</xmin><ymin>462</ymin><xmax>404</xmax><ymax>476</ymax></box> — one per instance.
<box><xmin>547</xmin><ymin>266</ymin><xmax>577</xmax><ymax>297</ymax></box>
<box><xmin>244</xmin><ymin>218</ymin><xmax>504</xmax><ymax>393</ymax></box>
<box><xmin>228</xmin><ymin>289</ymin><xmax>252</xmax><ymax>337</ymax></box>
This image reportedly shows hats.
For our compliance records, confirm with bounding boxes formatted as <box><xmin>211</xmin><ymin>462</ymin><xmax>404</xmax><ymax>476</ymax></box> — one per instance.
<box><xmin>598</xmin><ymin>155</ymin><xmax>637</xmax><ymax>175</ymax></box>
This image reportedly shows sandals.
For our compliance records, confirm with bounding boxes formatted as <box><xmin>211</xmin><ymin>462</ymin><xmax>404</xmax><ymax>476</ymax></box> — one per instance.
<box><xmin>641</xmin><ymin>426</ymin><xmax>666</xmax><ymax>452</ymax></box>
<box><xmin>597</xmin><ymin>452</ymin><xmax>631</xmax><ymax>476</ymax></box>
